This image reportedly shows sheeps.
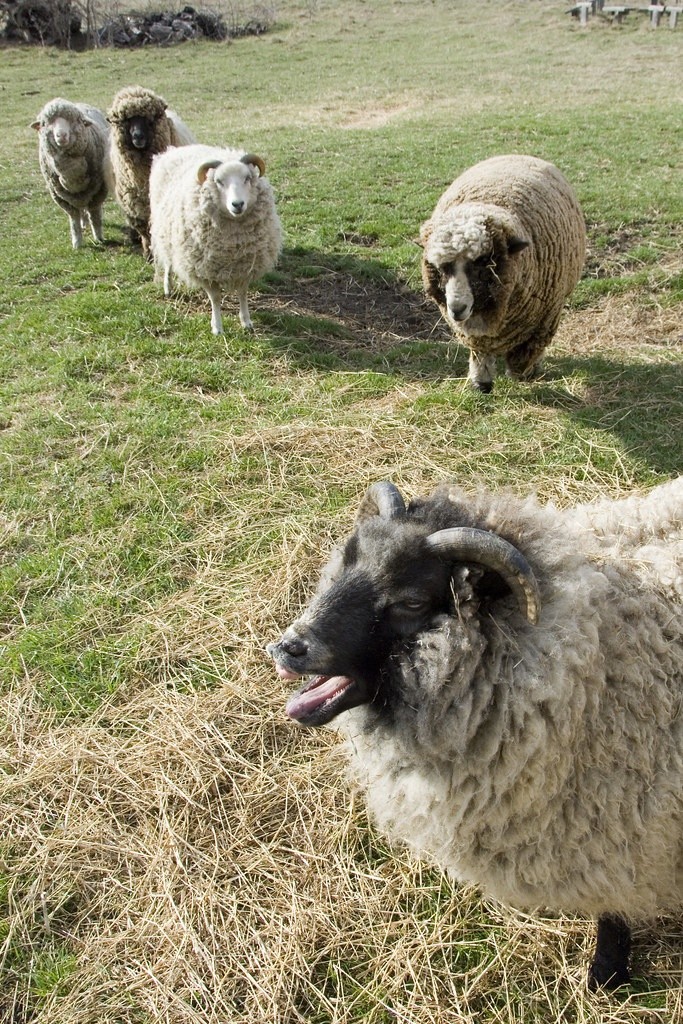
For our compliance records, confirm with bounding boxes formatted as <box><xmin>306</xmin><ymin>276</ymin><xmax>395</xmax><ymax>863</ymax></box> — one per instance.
<box><xmin>147</xmin><ymin>143</ymin><xmax>283</xmax><ymax>333</ymax></box>
<box><xmin>31</xmin><ymin>97</ymin><xmax>116</xmax><ymax>249</ymax></box>
<box><xmin>107</xmin><ymin>85</ymin><xmax>193</xmax><ymax>260</ymax></box>
<box><xmin>418</xmin><ymin>154</ymin><xmax>586</xmax><ymax>393</ymax></box>
<box><xmin>266</xmin><ymin>481</ymin><xmax>683</xmax><ymax>993</ymax></box>
